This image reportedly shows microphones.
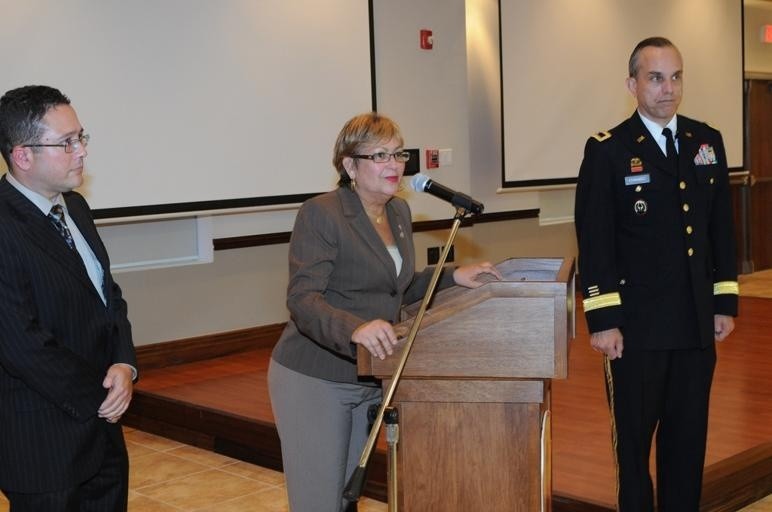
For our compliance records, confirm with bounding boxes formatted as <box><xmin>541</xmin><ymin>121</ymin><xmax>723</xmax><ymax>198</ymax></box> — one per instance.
<box><xmin>410</xmin><ymin>174</ymin><xmax>484</xmax><ymax>215</ymax></box>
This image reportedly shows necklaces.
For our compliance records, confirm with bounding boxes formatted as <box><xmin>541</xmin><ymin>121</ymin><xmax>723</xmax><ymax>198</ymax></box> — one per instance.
<box><xmin>363</xmin><ymin>205</ymin><xmax>389</xmax><ymax>223</ymax></box>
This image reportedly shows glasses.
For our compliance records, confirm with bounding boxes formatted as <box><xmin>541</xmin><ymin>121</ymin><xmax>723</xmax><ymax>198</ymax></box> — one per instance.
<box><xmin>351</xmin><ymin>151</ymin><xmax>411</xmax><ymax>164</ymax></box>
<box><xmin>10</xmin><ymin>134</ymin><xmax>89</xmax><ymax>153</ymax></box>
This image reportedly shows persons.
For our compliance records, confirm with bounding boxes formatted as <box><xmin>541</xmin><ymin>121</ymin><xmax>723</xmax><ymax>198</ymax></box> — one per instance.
<box><xmin>0</xmin><ymin>84</ymin><xmax>142</xmax><ymax>511</ymax></box>
<box><xmin>573</xmin><ymin>36</ymin><xmax>740</xmax><ymax>511</ymax></box>
<box><xmin>266</xmin><ymin>112</ymin><xmax>505</xmax><ymax>511</ymax></box>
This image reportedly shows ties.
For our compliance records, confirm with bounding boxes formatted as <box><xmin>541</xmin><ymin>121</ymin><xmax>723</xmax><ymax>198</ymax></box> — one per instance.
<box><xmin>662</xmin><ymin>127</ymin><xmax>679</xmax><ymax>166</ymax></box>
<box><xmin>48</xmin><ymin>204</ymin><xmax>76</xmax><ymax>254</ymax></box>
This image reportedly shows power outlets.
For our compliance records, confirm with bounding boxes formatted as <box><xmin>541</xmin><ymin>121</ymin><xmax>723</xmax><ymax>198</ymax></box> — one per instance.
<box><xmin>427</xmin><ymin>246</ymin><xmax>441</xmax><ymax>266</ymax></box>
<box><xmin>442</xmin><ymin>244</ymin><xmax>455</xmax><ymax>263</ymax></box>
